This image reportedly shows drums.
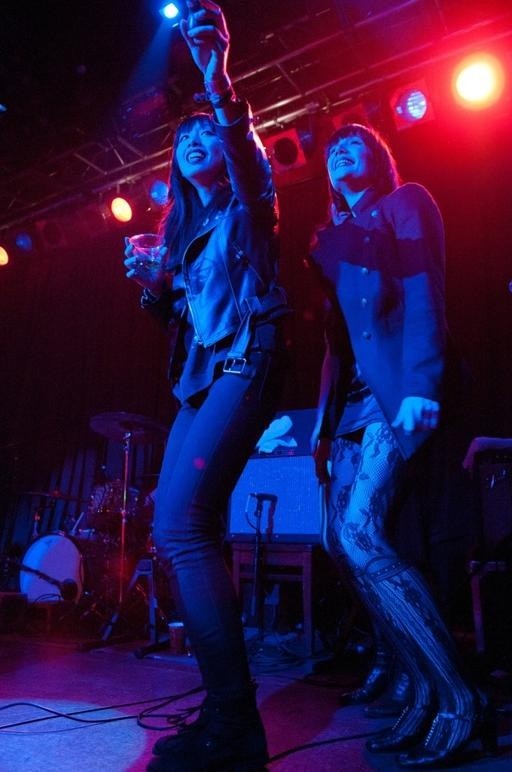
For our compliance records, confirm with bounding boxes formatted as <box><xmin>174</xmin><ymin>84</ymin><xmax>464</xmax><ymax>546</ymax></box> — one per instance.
<box><xmin>88</xmin><ymin>480</ymin><xmax>141</xmax><ymax>533</ymax></box>
<box><xmin>18</xmin><ymin>530</ymin><xmax>128</xmax><ymax>627</ymax></box>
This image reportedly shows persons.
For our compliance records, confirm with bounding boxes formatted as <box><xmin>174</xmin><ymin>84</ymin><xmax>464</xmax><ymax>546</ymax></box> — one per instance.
<box><xmin>123</xmin><ymin>0</ymin><xmax>298</xmax><ymax>772</ymax></box>
<box><xmin>303</xmin><ymin>121</ymin><xmax>499</xmax><ymax>772</ymax></box>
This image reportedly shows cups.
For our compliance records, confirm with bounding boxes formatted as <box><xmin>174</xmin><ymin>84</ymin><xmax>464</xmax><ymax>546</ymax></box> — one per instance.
<box><xmin>168</xmin><ymin>621</ymin><xmax>186</xmax><ymax>652</ymax></box>
<box><xmin>130</xmin><ymin>235</ymin><xmax>164</xmax><ymax>273</ymax></box>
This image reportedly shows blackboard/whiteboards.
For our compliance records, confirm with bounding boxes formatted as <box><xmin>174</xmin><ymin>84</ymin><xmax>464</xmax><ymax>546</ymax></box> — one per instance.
<box><xmin>226</xmin><ymin>456</ymin><xmax>322</xmax><ymax>542</ymax></box>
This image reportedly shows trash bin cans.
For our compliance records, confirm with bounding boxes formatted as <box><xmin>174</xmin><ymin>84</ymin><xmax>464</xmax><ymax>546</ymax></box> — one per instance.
<box><xmin>169</xmin><ymin>621</ymin><xmax>184</xmax><ymax>655</ymax></box>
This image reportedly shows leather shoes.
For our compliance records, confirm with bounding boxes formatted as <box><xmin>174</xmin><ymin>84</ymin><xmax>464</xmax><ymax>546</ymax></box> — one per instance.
<box><xmin>148</xmin><ymin>705</ymin><xmax>267</xmax><ymax>772</ymax></box>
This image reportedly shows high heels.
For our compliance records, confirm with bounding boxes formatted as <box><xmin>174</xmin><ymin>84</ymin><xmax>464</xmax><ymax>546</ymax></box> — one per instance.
<box><xmin>335</xmin><ymin>655</ymin><xmax>489</xmax><ymax>768</ymax></box>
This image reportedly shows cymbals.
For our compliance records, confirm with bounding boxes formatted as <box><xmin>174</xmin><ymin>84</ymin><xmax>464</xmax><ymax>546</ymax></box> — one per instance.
<box><xmin>18</xmin><ymin>489</ymin><xmax>85</xmax><ymax>504</ymax></box>
<box><xmin>88</xmin><ymin>411</ymin><xmax>166</xmax><ymax>444</ymax></box>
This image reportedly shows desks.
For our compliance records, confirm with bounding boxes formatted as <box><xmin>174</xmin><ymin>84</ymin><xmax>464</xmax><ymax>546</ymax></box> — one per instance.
<box><xmin>230</xmin><ymin>541</ymin><xmax>321</xmax><ymax>659</ymax></box>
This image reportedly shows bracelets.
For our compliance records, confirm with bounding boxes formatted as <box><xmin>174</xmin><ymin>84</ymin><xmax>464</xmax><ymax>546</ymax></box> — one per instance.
<box><xmin>210</xmin><ymin>86</ymin><xmax>235</xmax><ymax>108</ymax></box>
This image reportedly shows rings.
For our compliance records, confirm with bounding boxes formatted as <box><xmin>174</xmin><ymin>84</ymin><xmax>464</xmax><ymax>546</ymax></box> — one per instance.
<box><xmin>433</xmin><ymin>412</ymin><xmax>440</xmax><ymax>418</ymax></box>
<box><xmin>423</xmin><ymin>410</ymin><xmax>432</xmax><ymax>418</ymax></box>
